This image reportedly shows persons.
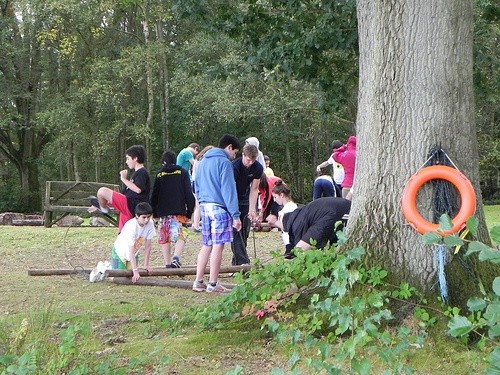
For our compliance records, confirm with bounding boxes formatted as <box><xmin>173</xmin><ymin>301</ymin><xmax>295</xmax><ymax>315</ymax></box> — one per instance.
<box><xmin>90</xmin><ymin>146</ymin><xmax>150</xmax><ymax>234</ymax></box>
<box><xmin>192</xmin><ymin>134</ymin><xmax>242</xmax><ymax>293</ymax></box>
<box><xmin>313</xmin><ymin>175</ymin><xmax>340</xmax><ymax>200</ymax></box>
<box><xmin>282</xmin><ymin>197</ymin><xmax>352</xmax><ymax>254</ymax></box>
<box><xmin>112</xmin><ymin>202</ymin><xmax>155</xmax><ymax>283</ymax></box>
<box><xmin>151</xmin><ymin>152</ymin><xmax>195</xmax><ymax>277</ymax></box>
<box><xmin>246</xmin><ymin>138</ymin><xmax>285</xmax><ymax>227</ymax></box>
<box><xmin>332</xmin><ymin>136</ymin><xmax>357</xmax><ymax>198</ymax></box>
<box><xmin>267</xmin><ymin>181</ymin><xmax>298</xmax><ymax>261</ymax></box>
<box><xmin>176</xmin><ymin>144</ymin><xmax>214</xmax><ymax>231</ymax></box>
<box><xmin>230</xmin><ymin>144</ymin><xmax>264</xmax><ymax>280</ymax></box>
<box><xmin>317</xmin><ymin>141</ymin><xmax>346</xmax><ymax>196</ymax></box>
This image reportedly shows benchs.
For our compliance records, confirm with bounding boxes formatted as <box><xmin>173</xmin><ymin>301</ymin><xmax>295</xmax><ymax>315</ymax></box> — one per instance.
<box><xmin>43</xmin><ymin>180</ymin><xmax>122</xmax><ymax>228</ymax></box>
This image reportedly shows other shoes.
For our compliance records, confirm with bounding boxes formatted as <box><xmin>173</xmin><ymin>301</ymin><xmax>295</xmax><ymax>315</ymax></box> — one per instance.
<box><xmin>192</xmin><ymin>279</ymin><xmax>208</xmax><ymax>291</ymax></box>
<box><xmin>205</xmin><ymin>282</ymin><xmax>232</xmax><ymax>293</ymax></box>
<box><xmin>90</xmin><ymin>260</ymin><xmax>111</xmax><ymax>283</ymax></box>
<box><xmin>89</xmin><ymin>198</ymin><xmax>110</xmax><ymax>214</ymax></box>
<box><xmin>165</xmin><ymin>263</ymin><xmax>171</xmax><ymax>278</ymax></box>
<box><xmin>194</xmin><ymin>227</ymin><xmax>201</xmax><ymax>232</ymax></box>
<box><xmin>171</xmin><ymin>260</ymin><xmax>186</xmax><ymax>278</ymax></box>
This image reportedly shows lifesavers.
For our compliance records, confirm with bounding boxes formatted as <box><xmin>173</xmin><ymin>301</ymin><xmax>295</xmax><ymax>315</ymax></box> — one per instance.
<box><xmin>400</xmin><ymin>164</ymin><xmax>476</xmax><ymax>237</ymax></box>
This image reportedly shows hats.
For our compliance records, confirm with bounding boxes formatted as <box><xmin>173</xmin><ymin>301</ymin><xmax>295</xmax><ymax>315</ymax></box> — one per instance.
<box><xmin>263</xmin><ymin>155</ymin><xmax>270</xmax><ymax>160</ymax></box>
